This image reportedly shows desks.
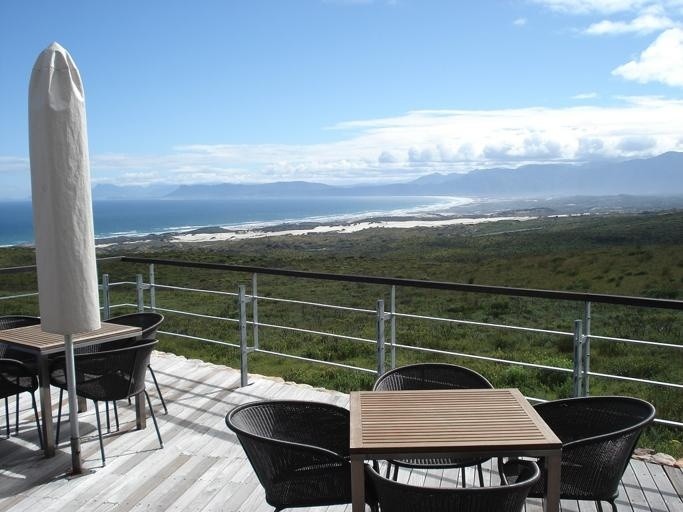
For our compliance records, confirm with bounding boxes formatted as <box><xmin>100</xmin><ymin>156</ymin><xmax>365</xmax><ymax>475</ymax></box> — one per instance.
<box><xmin>350</xmin><ymin>388</ymin><xmax>562</xmax><ymax>512</ymax></box>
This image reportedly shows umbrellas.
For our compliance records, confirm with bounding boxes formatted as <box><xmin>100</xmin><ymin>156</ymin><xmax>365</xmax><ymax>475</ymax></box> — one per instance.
<box><xmin>28</xmin><ymin>42</ymin><xmax>102</xmax><ymax>477</ymax></box>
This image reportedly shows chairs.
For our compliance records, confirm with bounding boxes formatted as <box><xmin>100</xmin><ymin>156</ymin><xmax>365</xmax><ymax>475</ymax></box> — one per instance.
<box><xmin>0</xmin><ymin>312</ymin><xmax>167</xmax><ymax>466</ymax></box>
<box><xmin>372</xmin><ymin>363</ymin><xmax>496</xmax><ymax>488</ymax></box>
<box><xmin>365</xmin><ymin>456</ymin><xmax>541</xmax><ymax>512</ymax></box>
<box><xmin>228</xmin><ymin>400</ymin><xmax>375</xmax><ymax>512</ymax></box>
<box><xmin>508</xmin><ymin>396</ymin><xmax>655</xmax><ymax>511</ymax></box>
